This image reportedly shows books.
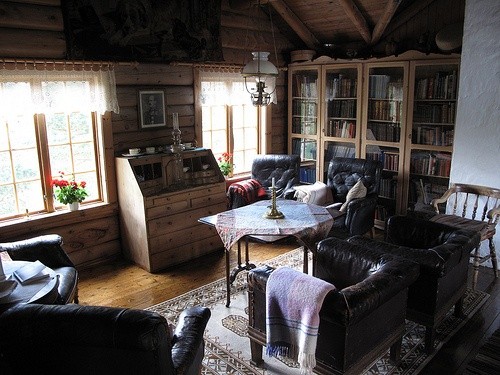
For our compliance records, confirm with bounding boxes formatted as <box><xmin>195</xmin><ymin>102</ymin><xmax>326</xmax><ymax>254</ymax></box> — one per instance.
<box><xmin>409</xmin><ymin>74</ymin><xmax>457</xmax><ymax>206</ymax></box>
<box><xmin>14</xmin><ymin>260</ymin><xmax>50</xmax><ymax>285</ymax></box>
<box><xmin>365</xmin><ymin>75</ymin><xmax>402</xmax><ymax>220</ymax></box>
<box><xmin>294</xmin><ymin>73</ymin><xmax>355</xmax><ymax>183</ymax></box>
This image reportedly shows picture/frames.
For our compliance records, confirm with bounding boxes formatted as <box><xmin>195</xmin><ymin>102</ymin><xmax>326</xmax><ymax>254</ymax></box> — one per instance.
<box><xmin>136</xmin><ymin>87</ymin><xmax>169</xmax><ymax>131</ymax></box>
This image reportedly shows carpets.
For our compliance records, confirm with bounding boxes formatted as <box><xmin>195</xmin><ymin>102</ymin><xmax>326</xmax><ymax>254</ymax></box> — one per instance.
<box><xmin>145</xmin><ymin>246</ymin><xmax>492</xmax><ymax>375</ymax></box>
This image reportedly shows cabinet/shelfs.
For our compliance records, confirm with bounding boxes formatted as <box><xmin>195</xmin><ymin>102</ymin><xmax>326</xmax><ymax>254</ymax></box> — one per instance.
<box><xmin>115</xmin><ymin>148</ymin><xmax>227</xmax><ymax>274</ymax></box>
<box><xmin>287</xmin><ymin>50</ymin><xmax>461</xmax><ymax>238</ymax></box>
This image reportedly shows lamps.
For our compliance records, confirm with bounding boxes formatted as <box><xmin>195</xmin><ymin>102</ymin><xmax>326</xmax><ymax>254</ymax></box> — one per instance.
<box><xmin>239</xmin><ymin>0</ymin><xmax>279</xmax><ymax>108</ymax></box>
<box><xmin>171</xmin><ymin>111</ymin><xmax>183</xmax><ymax>147</ymax></box>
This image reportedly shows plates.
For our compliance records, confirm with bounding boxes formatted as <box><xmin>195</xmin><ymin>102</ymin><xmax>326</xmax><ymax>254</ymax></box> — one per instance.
<box><xmin>185</xmin><ymin>147</ymin><xmax>196</xmax><ymax>149</ymax></box>
<box><xmin>122</xmin><ymin>153</ymin><xmax>144</xmax><ymax>156</ymax></box>
<box><xmin>142</xmin><ymin>152</ymin><xmax>158</xmax><ymax>154</ymax></box>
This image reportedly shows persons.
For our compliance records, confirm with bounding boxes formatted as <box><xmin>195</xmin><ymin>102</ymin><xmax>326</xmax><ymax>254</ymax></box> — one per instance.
<box><xmin>143</xmin><ymin>94</ymin><xmax>163</xmax><ymax>124</ymax></box>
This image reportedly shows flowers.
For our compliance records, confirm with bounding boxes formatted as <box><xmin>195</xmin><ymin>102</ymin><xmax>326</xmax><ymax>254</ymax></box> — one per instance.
<box><xmin>49</xmin><ymin>170</ymin><xmax>88</xmax><ymax>205</ymax></box>
<box><xmin>218</xmin><ymin>153</ymin><xmax>234</xmax><ymax>176</ymax></box>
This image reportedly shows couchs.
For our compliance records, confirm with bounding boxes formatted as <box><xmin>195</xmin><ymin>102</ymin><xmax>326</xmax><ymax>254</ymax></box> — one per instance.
<box><xmin>0</xmin><ymin>234</ymin><xmax>211</xmax><ymax>375</ymax></box>
<box><xmin>225</xmin><ymin>154</ymin><xmax>302</xmax><ymax>209</ymax></box>
<box><xmin>247</xmin><ymin>213</ymin><xmax>481</xmax><ymax>375</ymax></box>
<box><xmin>282</xmin><ymin>156</ymin><xmax>385</xmax><ymax>242</ymax></box>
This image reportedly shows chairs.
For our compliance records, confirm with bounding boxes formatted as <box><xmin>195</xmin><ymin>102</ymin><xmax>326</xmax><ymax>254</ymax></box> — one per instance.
<box><xmin>430</xmin><ymin>183</ymin><xmax>500</xmax><ymax>291</ymax></box>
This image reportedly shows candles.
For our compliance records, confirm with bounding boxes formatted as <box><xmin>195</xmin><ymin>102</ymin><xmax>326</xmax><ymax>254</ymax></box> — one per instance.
<box><xmin>272</xmin><ymin>176</ymin><xmax>275</xmax><ymax>186</ymax></box>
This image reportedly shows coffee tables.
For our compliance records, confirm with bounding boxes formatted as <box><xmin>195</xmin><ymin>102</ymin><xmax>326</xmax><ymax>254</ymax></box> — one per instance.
<box><xmin>0</xmin><ymin>259</ymin><xmax>59</xmax><ymax>311</ymax></box>
<box><xmin>198</xmin><ymin>197</ymin><xmax>345</xmax><ymax>308</ymax></box>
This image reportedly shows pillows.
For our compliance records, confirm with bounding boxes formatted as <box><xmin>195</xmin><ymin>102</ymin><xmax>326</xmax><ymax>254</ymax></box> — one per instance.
<box><xmin>339</xmin><ymin>177</ymin><xmax>368</xmax><ymax>213</ymax></box>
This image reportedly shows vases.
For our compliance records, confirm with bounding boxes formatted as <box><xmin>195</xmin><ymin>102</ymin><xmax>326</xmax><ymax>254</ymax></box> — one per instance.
<box><xmin>67</xmin><ymin>202</ymin><xmax>79</xmax><ymax>212</ymax></box>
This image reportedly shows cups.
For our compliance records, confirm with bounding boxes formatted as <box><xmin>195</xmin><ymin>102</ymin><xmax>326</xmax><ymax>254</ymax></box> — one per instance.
<box><xmin>203</xmin><ymin>165</ymin><xmax>208</xmax><ymax>169</ymax></box>
<box><xmin>183</xmin><ymin>167</ymin><xmax>190</xmax><ymax>172</ymax></box>
<box><xmin>190</xmin><ymin>137</ymin><xmax>198</xmax><ymax>148</ymax></box>
<box><xmin>184</xmin><ymin>143</ymin><xmax>193</xmax><ymax>147</ymax></box>
<box><xmin>129</xmin><ymin>149</ymin><xmax>141</xmax><ymax>154</ymax></box>
<box><xmin>146</xmin><ymin>147</ymin><xmax>155</xmax><ymax>153</ymax></box>
